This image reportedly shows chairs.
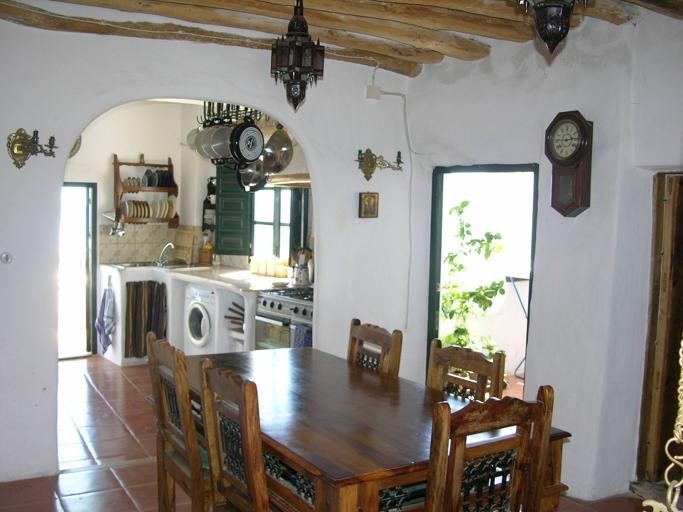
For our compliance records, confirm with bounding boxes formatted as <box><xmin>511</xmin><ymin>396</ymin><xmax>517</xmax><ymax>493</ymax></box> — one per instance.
<box><xmin>426</xmin><ymin>339</ymin><xmax>504</xmax><ymax>400</ymax></box>
<box><xmin>347</xmin><ymin>319</ymin><xmax>402</xmax><ymax>378</ymax></box>
<box><xmin>199</xmin><ymin>359</ymin><xmax>271</xmax><ymax>512</ymax></box>
<box><xmin>407</xmin><ymin>385</ymin><xmax>554</xmax><ymax>512</ymax></box>
<box><xmin>146</xmin><ymin>332</ymin><xmax>230</xmax><ymax>512</ymax></box>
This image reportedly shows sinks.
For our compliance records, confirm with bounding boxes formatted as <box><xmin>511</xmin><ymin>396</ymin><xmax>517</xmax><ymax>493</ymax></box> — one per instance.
<box><xmin>110</xmin><ymin>258</ymin><xmax>196</xmax><ymax>269</ymax></box>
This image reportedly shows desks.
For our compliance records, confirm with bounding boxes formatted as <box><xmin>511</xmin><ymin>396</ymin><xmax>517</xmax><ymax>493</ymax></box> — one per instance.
<box><xmin>144</xmin><ymin>346</ymin><xmax>572</xmax><ymax>512</ymax></box>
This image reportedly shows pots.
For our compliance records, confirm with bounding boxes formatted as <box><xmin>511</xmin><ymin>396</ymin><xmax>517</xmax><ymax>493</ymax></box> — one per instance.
<box><xmin>264</xmin><ymin>129</ymin><xmax>293</xmax><ymax>174</ymax></box>
<box><xmin>235</xmin><ymin>161</ymin><xmax>269</xmax><ymax>193</ymax></box>
<box><xmin>187</xmin><ymin>100</ymin><xmax>266</xmax><ymax>169</ymax></box>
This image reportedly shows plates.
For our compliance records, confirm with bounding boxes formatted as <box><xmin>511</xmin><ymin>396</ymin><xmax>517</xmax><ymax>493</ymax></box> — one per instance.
<box><xmin>127</xmin><ymin>169</ymin><xmax>174</xmax><ymax>193</ymax></box>
<box><xmin>123</xmin><ymin>193</ymin><xmax>178</xmax><ymax>218</ymax></box>
<box><xmin>272</xmin><ymin>282</ymin><xmax>289</xmax><ymax>286</ymax></box>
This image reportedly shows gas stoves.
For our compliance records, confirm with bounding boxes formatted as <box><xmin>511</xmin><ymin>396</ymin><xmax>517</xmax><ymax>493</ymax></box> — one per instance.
<box><xmin>255</xmin><ymin>287</ymin><xmax>314</xmax><ymax>322</ymax></box>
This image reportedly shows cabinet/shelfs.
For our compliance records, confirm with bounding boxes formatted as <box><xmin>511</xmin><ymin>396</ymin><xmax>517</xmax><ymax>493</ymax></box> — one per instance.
<box><xmin>102</xmin><ymin>154</ymin><xmax>180</xmax><ymax>235</ymax></box>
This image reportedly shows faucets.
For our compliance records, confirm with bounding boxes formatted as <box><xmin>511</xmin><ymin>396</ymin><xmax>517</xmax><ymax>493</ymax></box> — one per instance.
<box><xmin>152</xmin><ymin>242</ymin><xmax>175</xmax><ymax>267</ymax></box>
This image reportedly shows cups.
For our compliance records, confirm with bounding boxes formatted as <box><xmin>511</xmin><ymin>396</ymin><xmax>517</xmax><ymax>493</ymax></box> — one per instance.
<box><xmin>205</xmin><ymin>194</ymin><xmax>217</xmax><ymax>201</ymax></box>
<box><xmin>249</xmin><ymin>256</ymin><xmax>288</xmax><ymax>277</ymax></box>
<box><xmin>211</xmin><ymin>252</ymin><xmax>221</xmax><ymax>265</ymax></box>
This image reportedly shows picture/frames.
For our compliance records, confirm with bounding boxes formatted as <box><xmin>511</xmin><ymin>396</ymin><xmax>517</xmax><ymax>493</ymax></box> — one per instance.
<box><xmin>359</xmin><ymin>192</ymin><xmax>379</xmax><ymax>219</ymax></box>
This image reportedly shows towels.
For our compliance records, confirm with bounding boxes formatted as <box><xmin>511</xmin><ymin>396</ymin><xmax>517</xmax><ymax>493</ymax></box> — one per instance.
<box><xmin>94</xmin><ymin>287</ymin><xmax>115</xmax><ymax>354</ymax></box>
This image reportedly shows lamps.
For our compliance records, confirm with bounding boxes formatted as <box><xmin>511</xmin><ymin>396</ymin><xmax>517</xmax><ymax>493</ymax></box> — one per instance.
<box><xmin>7</xmin><ymin>128</ymin><xmax>59</xmax><ymax>170</ymax></box>
<box><xmin>271</xmin><ymin>0</ymin><xmax>325</xmax><ymax>113</ymax></box>
<box><xmin>516</xmin><ymin>0</ymin><xmax>587</xmax><ymax>54</ymax></box>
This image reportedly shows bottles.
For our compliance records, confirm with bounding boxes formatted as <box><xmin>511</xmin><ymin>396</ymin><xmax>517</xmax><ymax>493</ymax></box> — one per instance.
<box><xmin>202</xmin><ymin>233</ymin><xmax>214</xmax><ymax>251</ymax></box>
<box><xmin>293</xmin><ymin>263</ymin><xmax>309</xmax><ymax>286</ymax></box>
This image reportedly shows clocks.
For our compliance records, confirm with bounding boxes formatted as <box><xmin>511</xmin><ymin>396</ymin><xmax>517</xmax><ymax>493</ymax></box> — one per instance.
<box><xmin>544</xmin><ymin>110</ymin><xmax>593</xmax><ymax>217</ymax></box>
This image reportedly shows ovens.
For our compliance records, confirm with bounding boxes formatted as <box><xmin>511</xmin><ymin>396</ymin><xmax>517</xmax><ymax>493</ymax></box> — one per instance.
<box><xmin>252</xmin><ymin>313</ymin><xmax>313</xmax><ymax>348</ymax></box>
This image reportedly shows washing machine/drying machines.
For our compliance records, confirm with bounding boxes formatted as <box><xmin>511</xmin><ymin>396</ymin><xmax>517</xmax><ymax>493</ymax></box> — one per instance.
<box><xmin>183</xmin><ymin>284</ymin><xmax>215</xmax><ymax>356</ymax></box>
